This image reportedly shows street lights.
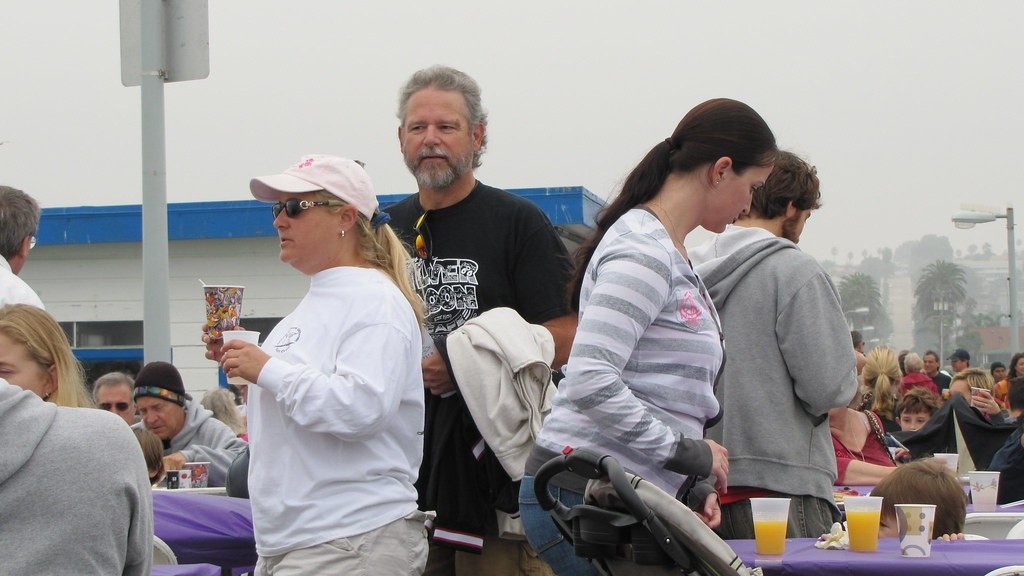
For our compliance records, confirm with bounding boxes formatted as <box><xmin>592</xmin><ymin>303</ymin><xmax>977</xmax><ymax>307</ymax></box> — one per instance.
<box><xmin>951</xmin><ymin>208</ymin><xmax>1020</xmax><ymax>355</ymax></box>
<box><xmin>939</xmin><ymin>321</ymin><xmax>948</xmax><ymax>367</ymax></box>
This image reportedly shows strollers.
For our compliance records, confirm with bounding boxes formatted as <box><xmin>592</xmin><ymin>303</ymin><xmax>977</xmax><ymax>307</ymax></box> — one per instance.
<box><xmin>533</xmin><ymin>445</ymin><xmax>766</xmax><ymax>576</ymax></box>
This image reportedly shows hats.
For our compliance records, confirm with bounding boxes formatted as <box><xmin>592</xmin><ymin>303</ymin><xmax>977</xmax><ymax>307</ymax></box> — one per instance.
<box><xmin>133</xmin><ymin>361</ymin><xmax>186</xmax><ymax>407</ymax></box>
<box><xmin>250</xmin><ymin>153</ymin><xmax>379</xmax><ymax>221</ymax></box>
<box><xmin>946</xmin><ymin>348</ymin><xmax>971</xmax><ymax>361</ymax></box>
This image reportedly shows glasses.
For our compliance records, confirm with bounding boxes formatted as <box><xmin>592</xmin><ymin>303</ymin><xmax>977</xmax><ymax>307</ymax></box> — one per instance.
<box><xmin>271</xmin><ymin>198</ymin><xmax>363</xmax><ymax>227</ymax></box>
<box><xmin>413</xmin><ymin>209</ymin><xmax>434</xmax><ymax>264</ymax></box>
<box><xmin>30</xmin><ymin>236</ymin><xmax>36</xmax><ymax>249</ymax></box>
<box><xmin>98</xmin><ymin>400</ymin><xmax>130</xmax><ymax>412</ymax></box>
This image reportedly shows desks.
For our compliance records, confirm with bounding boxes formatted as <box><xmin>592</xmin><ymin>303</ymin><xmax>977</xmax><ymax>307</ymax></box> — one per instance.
<box><xmin>152</xmin><ymin>488</ymin><xmax>258</xmax><ymax>576</ymax></box>
<box><xmin>721</xmin><ymin>485</ymin><xmax>1024</xmax><ymax>576</ymax></box>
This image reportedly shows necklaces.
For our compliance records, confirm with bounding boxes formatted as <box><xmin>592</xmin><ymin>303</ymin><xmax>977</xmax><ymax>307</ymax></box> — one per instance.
<box><xmin>648</xmin><ymin>200</ymin><xmax>687</xmax><ymax>253</ymax></box>
<box><xmin>834</xmin><ymin>411</ymin><xmax>866</xmax><ymax>464</ymax></box>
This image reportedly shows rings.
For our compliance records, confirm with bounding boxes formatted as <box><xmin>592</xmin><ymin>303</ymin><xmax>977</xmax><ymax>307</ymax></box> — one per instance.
<box><xmin>984</xmin><ymin>403</ymin><xmax>987</xmax><ymax>408</ymax></box>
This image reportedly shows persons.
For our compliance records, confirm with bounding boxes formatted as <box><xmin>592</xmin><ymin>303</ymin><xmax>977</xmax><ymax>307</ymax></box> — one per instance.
<box><xmin>687</xmin><ymin>149</ymin><xmax>858</xmax><ymax>542</ymax></box>
<box><xmin>826</xmin><ymin>330</ymin><xmax>1024</xmax><ymax>505</ymax></box>
<box><xmin>0</xmin><ymin>301</ymin><xmax>97</xmax><ymax>408</ymax></box>
<box><xmin>92</xmin><ymin>371</ymin><xmax>137</xmax><ymax>426</ymax></box>
<box><xmin>132</xmin><ymin>359</ymin><xmax>250</xmax><ymax>499</ymax></box>
<box><xmin>518</xmin><ymin>99</ymin><xmax>778</xmax><ymax>576</ymax></box>
<box><xmin>0</xmin><ymin>377</ymin><xmax>154</xmax><ymax>576</ymax></box>
<box><xmin>0</xmin><ymin>185</ymin><xmax>45</xmax><ymax>314</ymax></box>
<box><xmin>382</xmin><ymin>61</ymin><xmax>578</xmax><ymax>576</ymax></box>
<box><xmin>868</xmin><ymin>459</ymin><xmax>969</xmax><ymax>542</ymax></box>
<box><xmin>202</xmin><ymin>155</ymin><xmax>438</xmax><ymax>576</ymax></box>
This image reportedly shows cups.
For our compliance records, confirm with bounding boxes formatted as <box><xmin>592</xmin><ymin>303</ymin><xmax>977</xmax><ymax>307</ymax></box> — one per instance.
<box><xmin>749</xmin><ymin>498</ymin><xmax>792</xmax><ymax>555</ymax></box>
<box><xmin>843</xmin><ymin>496</ymin><xmax>884</xmax><ymax>552</ymax></box>
<box><xmin>933</xmin><ymin>454</ymin><xmax>959</xmax><ymax>477</ymax></box>
<box><xmin>221</xmin><ymin>330</ymin><xmax>261</xmax><ymax>385</ymax></box>
<box><xmin>967</xmin><ymin>471</ymin><xmax>1000</xmax><ymax>511</ymax></box>
<box><xmin>202</xmin><ymin>285</ymin><xmax>245</xmax><ymax>339</ymax></box>
<box><xmin>894</xmin><ymin>503</ymin><xmax>937</xmax><ymax>557</ymax></box>
<box><xmin>184</xmin><ymin>462</ymin><xmax>210</xmax><ymax>488</ymax></box>
<box><xmin>970</xmin><ymin>387</ymin><xmax>991</xmax><ymax>416</ymax></box>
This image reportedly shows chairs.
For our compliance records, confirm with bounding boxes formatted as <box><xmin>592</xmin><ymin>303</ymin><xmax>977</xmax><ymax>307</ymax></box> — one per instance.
<box><xmin>963</xmin><ymin>513</ymin><xmax>1024</xmax><ymax>540</ymax></box>
